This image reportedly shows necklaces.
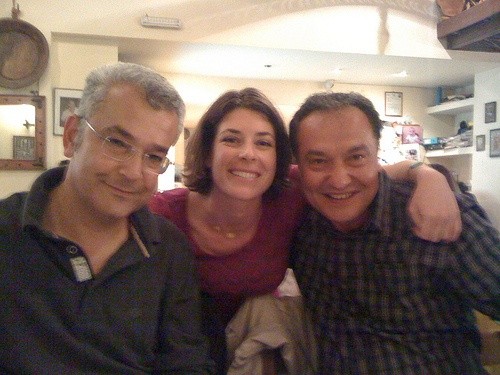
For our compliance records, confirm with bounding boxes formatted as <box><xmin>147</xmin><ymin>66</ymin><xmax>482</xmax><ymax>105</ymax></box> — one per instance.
<box><xmin>202</xmin><ymin>202</ymin><xmax>261</xmax><ymax>237</ymax></box>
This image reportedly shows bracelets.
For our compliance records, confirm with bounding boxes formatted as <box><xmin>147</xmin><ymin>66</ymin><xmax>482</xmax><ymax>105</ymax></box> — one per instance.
<box><xmin>408</xmin><ymin>161</ymin><xmax>426</xmax><ymax>181</ymax></box>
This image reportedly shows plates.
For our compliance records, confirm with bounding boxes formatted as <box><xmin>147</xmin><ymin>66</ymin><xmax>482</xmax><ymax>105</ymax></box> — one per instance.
<box><xmin>0</xmin><ymin>16</ymin><xmax>50</xmax><ymax>89</ymax></box>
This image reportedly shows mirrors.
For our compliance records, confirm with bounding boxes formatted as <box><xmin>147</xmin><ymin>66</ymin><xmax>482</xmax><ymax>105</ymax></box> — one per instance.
<box><xmin>0</xmin><ymin>94</ymin><xmax>46</xmax><ymax>170</ymax></box>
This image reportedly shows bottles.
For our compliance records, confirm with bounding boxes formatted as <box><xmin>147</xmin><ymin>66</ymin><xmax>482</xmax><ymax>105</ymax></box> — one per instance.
<box><xmin>434</xmin><ymin>87</ymin><xmax>442</xmax><ymax>106</ymax></box>
<box><xmin>458</xmin><ymin>120</ymin><xmax>471</xmax><ymax>147</ymax></box>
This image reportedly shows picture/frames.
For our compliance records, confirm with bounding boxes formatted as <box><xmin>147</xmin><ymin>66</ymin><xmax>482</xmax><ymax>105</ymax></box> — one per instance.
<box><xmin>476</xmin><ymin>135</ymin><xmax>485</xmax><ymax>151</ymax></box>
<box><xmin>485</xmin><ymin>102</ymin><xmax>496</xmax><ymax>123</ymax></box>
<box><xmin>489</xmin><ymin>128</ymin><xmax>500</xmax><ymax>157</ymax></box>
<box><xmin>402</xmin><ymin>125</ymin><xmax>423</xmax><ymax>144</ymax></box>
<box><xmin>53</xmin><ymin>88</ymin><xmax>84</xmax><ymax>136</ymax></box>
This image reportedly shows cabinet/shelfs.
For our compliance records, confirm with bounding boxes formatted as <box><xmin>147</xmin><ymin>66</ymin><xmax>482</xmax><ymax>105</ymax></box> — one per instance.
<box><xmin>425</xmin><ymin>97</ymin><xmax>474</xmax><ymax>159</ymax></box>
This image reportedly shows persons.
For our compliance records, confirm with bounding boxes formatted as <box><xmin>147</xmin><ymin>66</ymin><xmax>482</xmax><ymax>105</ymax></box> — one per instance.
<box><xmin>0</xmin><ymin>62</ymin><xmax>216</xmax><ymax>374</ymax></box>
<box><xmin>147</xmin><ymin>87</ymin><xmax>462</xmax><ymax>375</ymax></box>
<box><xmin>289</xmin><ymin>92</ymin><xmax>500</xmax><ymax>374</ymax></box>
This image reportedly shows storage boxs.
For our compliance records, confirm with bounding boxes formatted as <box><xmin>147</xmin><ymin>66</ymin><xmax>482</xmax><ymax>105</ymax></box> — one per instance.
<box><xmin>423</xmin><ymin>137</ymin><xmax>439</xmax><ymax>145</ymax></box>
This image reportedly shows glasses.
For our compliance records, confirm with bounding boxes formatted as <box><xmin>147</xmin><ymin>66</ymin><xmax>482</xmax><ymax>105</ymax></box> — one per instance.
<box><xmin>81</xmin><ymin>115</ymin><xmax>170</xmax><ymax>175</ymax></box>
